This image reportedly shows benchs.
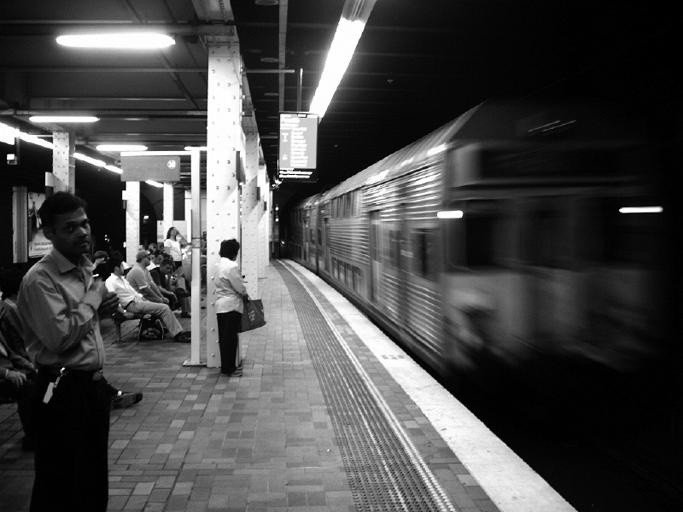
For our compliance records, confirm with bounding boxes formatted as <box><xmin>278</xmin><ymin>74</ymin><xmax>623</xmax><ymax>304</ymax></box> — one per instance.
<box><xmin>97</xmin><ymin>291</ymin><xmax>164</xmax><ymax>345</ymax></box>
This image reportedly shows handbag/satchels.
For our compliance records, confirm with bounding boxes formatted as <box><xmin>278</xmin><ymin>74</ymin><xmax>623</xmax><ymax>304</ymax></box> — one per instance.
<box><xmin>238</xmin><ymin>293</ymin><xmax>266</xmax><ymax>333</ymax></box>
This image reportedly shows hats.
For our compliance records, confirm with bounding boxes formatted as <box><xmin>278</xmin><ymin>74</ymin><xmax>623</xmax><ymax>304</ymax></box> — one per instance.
<box><xmin>135</xmin><ymin>249</ymin><xmax>150</xmax><ymax>260</ymax></box>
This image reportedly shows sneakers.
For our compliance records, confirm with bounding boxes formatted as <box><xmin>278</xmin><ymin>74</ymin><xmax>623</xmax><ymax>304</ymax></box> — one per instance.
<box><xmin>232</xmin><ymin>366</ymin><xmax>243</xmax><ymax>377</ymax></box>
<box><xmin>172</xmin><ymin>330</ymin><xmax>191</xmax><ymax>343</ymax></box>
<box><xmin>111</xmin><ymin>391</ymin><xmax>143</xmax><ymax>409</ymax></box>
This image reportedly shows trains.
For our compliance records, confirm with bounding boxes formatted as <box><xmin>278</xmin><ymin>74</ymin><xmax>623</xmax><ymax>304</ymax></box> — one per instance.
<box><xmin>281</xmin><ymin>89</ymin><xmax>680</xmax><ymax>387</ymax></box>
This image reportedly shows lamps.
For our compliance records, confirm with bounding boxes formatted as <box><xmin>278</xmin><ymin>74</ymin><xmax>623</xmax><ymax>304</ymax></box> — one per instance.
<box><xmin>6</xmin><ymin>135</ymin><xmax>21</xmax><ymax>166</ymax></box>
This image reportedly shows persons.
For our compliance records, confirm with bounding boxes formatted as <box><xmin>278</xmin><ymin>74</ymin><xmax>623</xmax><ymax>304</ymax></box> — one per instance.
<box><xmin>89</xmin><ymin>225</ymin><xmax>191</xmax><ymax>344</ymax></box>
<box><xmin>214</xmin><ymin>238</ymin><xmax>247</xmax><ymax>377</ymax></box>
<box><xmin>0</xmin><ymin>333</ymin><xmax>39</xmax><ymax>450</ymax></box>
<box><xmin>14</xmin><ymin>190</ymin><xmax>114</xmax><ymax>512</ymax></box>
<box><xmin>0</xmin><ymin>291</ymin><xmax>143</xmax><ymax>407</ymax></box>
